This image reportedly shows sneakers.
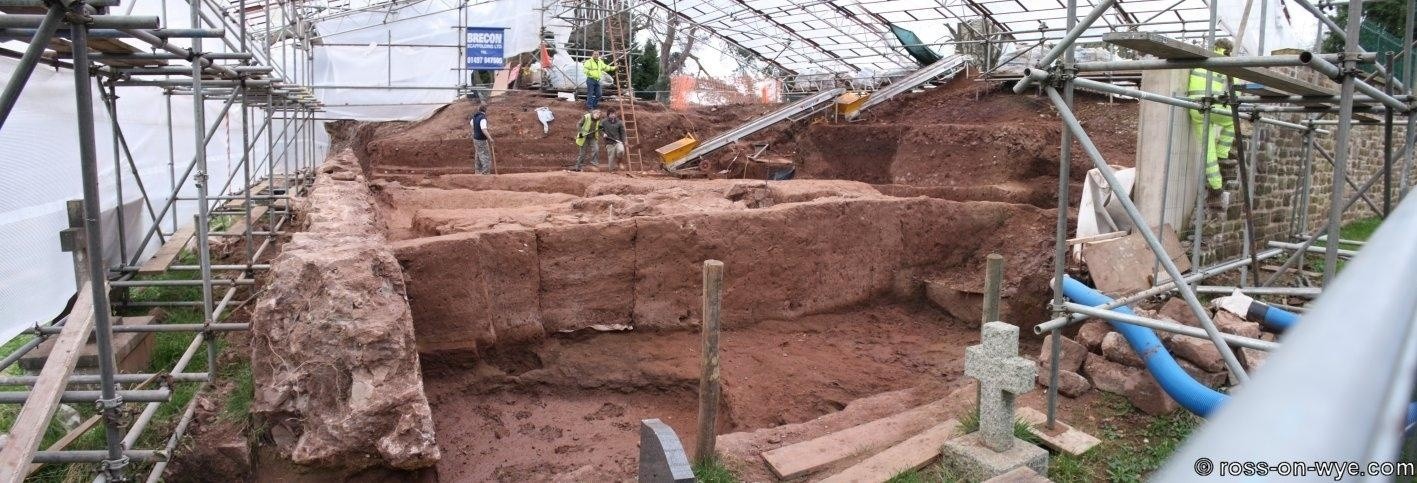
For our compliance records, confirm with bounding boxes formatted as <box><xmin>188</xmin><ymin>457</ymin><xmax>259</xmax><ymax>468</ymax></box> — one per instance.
<box><xmin>1211</xmin><ymin>181</ymin><xmax>1224</xmax><ymax>196</ymax></box>
<box><xmin>590</xmin><ymin>160</ymin><xmax>626</xmax><ymax>173</ymax></box>
<box><xmin>1218</xmin><ymin>157</ymin><xmax>1238</xmax><ymax>168</ymax></box>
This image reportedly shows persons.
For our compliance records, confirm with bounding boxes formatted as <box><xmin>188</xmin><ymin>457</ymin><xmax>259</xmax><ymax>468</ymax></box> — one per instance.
<box><xmin>598</xmin><ymin>105</ymin><xmax>626</xmax><ymax>171</ymax></box>
<box><xmin>468</xmin><ymin>105</ymin><xmax>494</xmax><ymax>174</ymax></box>
<box><xmin>1187</xmin><ymin>37</ymin><xmax>1240</xmax><ymax>195</ymax></box>
<box><xmin>574</xmin><ymin>108</ymin><xmax>601</xmax><ymax>171</ymax></box>
<box><xmin>583</xmin><ymin>47</ymin><xmax>620</xmax><ymax>108</ymax></box>
